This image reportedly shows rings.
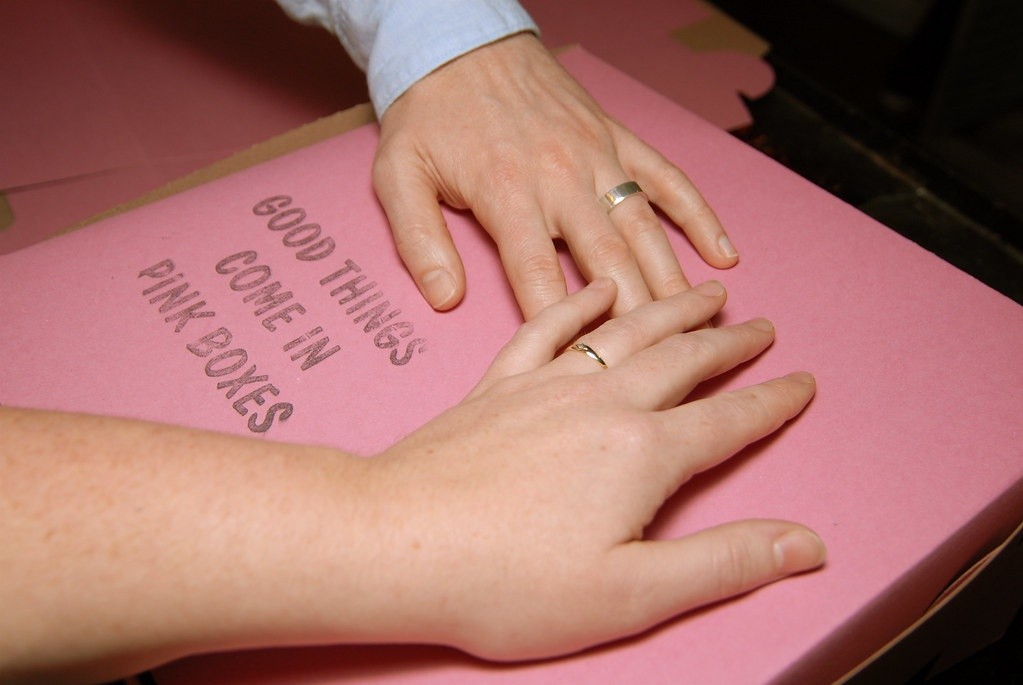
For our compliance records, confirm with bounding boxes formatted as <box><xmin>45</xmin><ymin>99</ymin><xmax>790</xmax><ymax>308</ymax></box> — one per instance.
<box><xmin>564</xmin><ymin>342</ymin><xmax>611</xmax><ymax>369</ymax></box>
<box><xmin>600</xmin><ymin>181</ymin><xmax>652</xmax><ymax>213</ymax></box>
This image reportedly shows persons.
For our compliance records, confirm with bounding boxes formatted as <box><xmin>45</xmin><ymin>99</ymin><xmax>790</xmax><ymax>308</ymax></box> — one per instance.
<box><xmin>277</xmin><ymin>0</ymin><xmax>742</xmax><ymax>351</ymax></box>
<box><xmin>0</xmin><ymin>276</ymin><xmax>829</xmax><ymax>685</ymax></box>
